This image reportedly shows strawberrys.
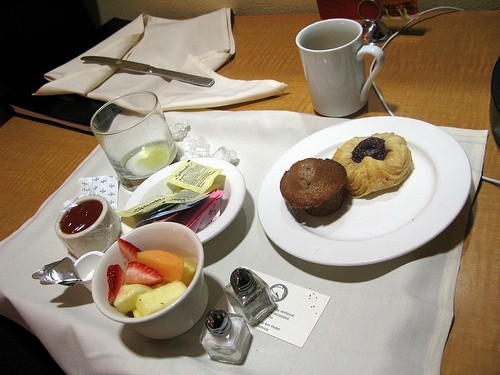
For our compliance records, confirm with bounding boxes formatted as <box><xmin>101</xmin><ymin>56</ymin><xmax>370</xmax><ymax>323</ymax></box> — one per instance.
<box><xmin>107</xmin><ymin>264</ymin><xmax>124</xmax><ymax>306</ymax></box>
<box><xmin>117</xmin><ymin>238</ymin><xmax>141</xmax><ymax>262</ymax></box>
<box><xmin>123</xmin><ymin>262</ymin><xmax>164</xmax><ymax>286</ymax></box>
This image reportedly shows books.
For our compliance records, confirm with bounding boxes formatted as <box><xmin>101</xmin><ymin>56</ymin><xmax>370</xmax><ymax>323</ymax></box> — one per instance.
<box><xmin>7</xmin><ymin>15</ymin><xmax>133</xmax><ymax>137</ymax></box>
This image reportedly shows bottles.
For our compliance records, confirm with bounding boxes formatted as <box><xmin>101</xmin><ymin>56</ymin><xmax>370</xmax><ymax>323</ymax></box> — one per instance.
<box><xmin>224</xmin><ymin>267</ymin><xmax>278</xmax><ymax>327</ymax></box>
<box><xmin>198</xmin><ymin>309</ymin><xmax>253</xmax><ymax>366</ymax></box>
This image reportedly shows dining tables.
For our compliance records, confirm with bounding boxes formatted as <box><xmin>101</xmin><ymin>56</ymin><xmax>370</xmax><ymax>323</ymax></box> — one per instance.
<box><xmin>0</xmin><ymin>11</ymin><xmax>500</xmax><ymax>375</ymax></box>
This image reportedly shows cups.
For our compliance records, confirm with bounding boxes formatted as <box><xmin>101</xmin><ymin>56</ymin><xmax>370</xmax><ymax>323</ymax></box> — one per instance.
<box><xmin>55</xmin><ymin>196</ymin><xmax>122</xmax><ymax>258</ymax></box>
<box><xmin>73</xmin><ymin>250</ymin><xmax>103</xmax><ymax>295</ymax></box>
<box><xmin>90</xmin><ymin>223</ymin><xmax>210</xmax><ymax>340</ymax></box>
<box><xmin>88</xmin><ymin>88</ymin><xmax>179</xmax><ymax>192</ymax></box>
<box><xmin>294</xmin><ymin>19</ymin><xmax>385</xmax><ymax>117</ymax></box>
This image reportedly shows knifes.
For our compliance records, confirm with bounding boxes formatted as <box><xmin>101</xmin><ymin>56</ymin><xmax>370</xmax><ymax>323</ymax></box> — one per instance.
<box><xmin>80</xmin><ymin>54</ymin><xmax>215</xmax><ymax>89</ymax></box>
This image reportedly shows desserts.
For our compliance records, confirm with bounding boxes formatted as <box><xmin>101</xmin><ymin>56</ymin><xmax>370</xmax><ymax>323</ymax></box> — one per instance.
<box><xmin>330</xmin><ymin>132</ymin><xmax>413</xmax><ymax>197</ymax></box>
<box><xmin>280</xmin><ymin>158</ymin><xmax>347</xmax><ymax>217</ymax></box>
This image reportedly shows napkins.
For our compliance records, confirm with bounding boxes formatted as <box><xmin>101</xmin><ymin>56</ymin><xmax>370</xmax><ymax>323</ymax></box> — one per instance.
<box><xmin>0</xmin><ymin>110</ymin><xmax>489</xmax><ymax>375</ymax></box>
<box><xmin>31</xmin><ymin>7</ymin><xmax>288</xmax><ymax>115</ymax></box>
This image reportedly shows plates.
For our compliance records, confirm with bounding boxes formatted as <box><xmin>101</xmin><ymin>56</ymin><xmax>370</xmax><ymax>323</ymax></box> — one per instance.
<box><xmin>120</xmin><ymin>156</ymin><xmax>246</xmax><ymax>246</ymax></box>
<box><xmin>258</xmin><ymin>115</ymin><xmax>473</xmax><ymax>266</ymax></box>
